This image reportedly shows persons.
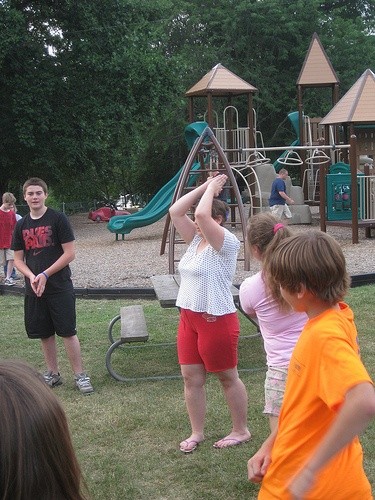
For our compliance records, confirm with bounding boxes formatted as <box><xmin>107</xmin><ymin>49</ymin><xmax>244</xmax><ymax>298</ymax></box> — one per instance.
<box><xmin>168</xmin><ymin>174</ymin><xmax>252</xmax><ymax>452</ymax></box>
<box><xmin>248</xmin><ymin>231</ymin><xmax>375</xmax><ymax>500</ymax></box>
<box><xmin>0</xmin><ymin>192</ymin><xmax>24</xmax><ymax>286</ymax></box>
<box><xmin>11</xmin><ymin>177</ymin><xmax>93</xmax><ymax>393</ymax></box>
<box><xmin>0</xmin><ymin>358</ymin><xmax>82</xmax><ymax>500</ymax></box>
<box><xmin>239</xmin><ymin>214</ymin><xmax>310</xmax><ymax>434</ymax></box>
<box><xmin>269</xmin><ymin>169</ymin><xmax>295</xmax><ymax>225</ymax></box>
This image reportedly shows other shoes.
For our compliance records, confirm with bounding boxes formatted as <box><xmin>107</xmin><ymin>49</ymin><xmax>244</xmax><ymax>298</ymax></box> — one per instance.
<box><xmin>0</xmin><ymin>276</ymin><xmax>6</xmax><ymax>286</ymax></box>
<box><xmin>4</xmin><ymin>278</ymin><xmax>16</xmax><ymax>286</ymax></box>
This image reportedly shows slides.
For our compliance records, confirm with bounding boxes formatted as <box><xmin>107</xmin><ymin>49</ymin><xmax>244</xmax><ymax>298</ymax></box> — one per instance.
<box><xmin>107</xmin><ymin>150</ymin><xmax>210</xmax><ymax>240</ymax></box>
<box><xmin>227</xmin><ymin>140</ymin><xmax>299</xmax><ymax>204</ymax></box>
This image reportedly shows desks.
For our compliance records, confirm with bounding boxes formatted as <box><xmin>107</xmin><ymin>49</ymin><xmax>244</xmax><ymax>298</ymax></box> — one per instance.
<box><xmin>106</xmin><ymin>274</ymin><xmax>269</xmax><ymax>382</ymax></box>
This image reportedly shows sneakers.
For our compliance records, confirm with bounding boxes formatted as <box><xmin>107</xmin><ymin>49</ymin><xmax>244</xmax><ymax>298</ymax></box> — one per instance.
<box><xmin>43</xmin><ymin>371</ymin><xmax>62</xmax><ymax>388</ymax></box>
<box><xmin>75</xmin><ymin>373</ymin><xmax>93</xmax><ymax>393</ymax></box>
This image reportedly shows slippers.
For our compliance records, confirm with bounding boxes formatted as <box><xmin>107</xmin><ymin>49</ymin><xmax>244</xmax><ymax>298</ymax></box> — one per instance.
<box><xmin>213</xmin><ymin>437</ymin><xmax>253</xmax><ymax>448</ymax></box>
<box><xmin>180</xmin><ymin>439</ymin><xmax>205</xmax><ymax>453</ymax></box>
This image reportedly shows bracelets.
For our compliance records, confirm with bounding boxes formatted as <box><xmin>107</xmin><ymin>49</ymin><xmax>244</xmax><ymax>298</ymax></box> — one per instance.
<box><xmin>41</xmin><ymin>272</ymin><xmax>48</xmax><ymax>279</ymax></box>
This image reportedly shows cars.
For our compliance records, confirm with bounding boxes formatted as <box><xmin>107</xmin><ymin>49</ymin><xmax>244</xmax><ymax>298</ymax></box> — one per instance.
<box><xmin>87</xmin><ymin>199</ymin><xmax>140</xmax><ymax>223</ymax></box>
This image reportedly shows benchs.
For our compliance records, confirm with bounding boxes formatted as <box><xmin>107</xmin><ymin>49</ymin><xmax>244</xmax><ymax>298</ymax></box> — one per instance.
<box><xmin>121</xmin><ymin>305</ymin><xmax>149</xmax><ymax>344</ymax></box>
